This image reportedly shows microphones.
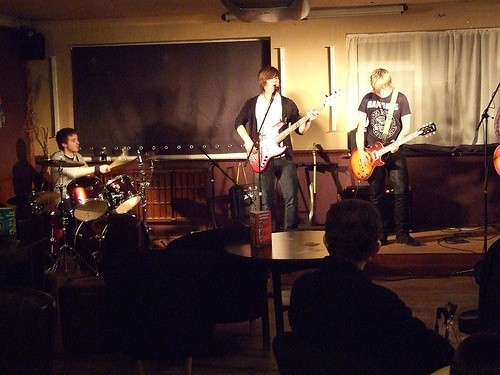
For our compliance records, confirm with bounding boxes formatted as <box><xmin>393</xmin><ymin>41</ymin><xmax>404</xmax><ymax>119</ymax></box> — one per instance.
<box><xmin>270</xmin><ymin>86</ymin><xmax>279</xmax><ymax>104</ymax></box>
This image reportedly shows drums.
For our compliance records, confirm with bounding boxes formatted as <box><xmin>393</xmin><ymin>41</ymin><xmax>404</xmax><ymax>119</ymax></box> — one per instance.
<box><xmin>74</xmin><ymin>213</ymin><xmax>150</xmax><ymax>275</ymax></box>
<box><xmin>104</xmin><ymin>174</ymin><xmax>141</xmax><ymax>214</ymax></box>
<box><xmin>66</xmin><ymin>175</ymin><xmax>108</xmax><ymax>221</ymax></box>
<box><xmin>229</xmin><ymin>184</ymin><xmax>261</xmax><ymax>229</ymax></box>
<box><xmin>45</xmin><ymin>210</ymin><xmax>70</xmax><ymax>243</ymax></box>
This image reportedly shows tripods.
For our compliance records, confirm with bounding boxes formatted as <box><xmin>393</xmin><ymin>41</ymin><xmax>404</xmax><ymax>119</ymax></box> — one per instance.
<box><xmin>44</xmin><ymin>163</ymin><xmax>99</xmax><ymax>277</ymax></box>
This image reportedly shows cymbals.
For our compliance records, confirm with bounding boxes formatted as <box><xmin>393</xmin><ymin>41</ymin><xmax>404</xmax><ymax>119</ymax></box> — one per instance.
<box><xmin>133</xmin><ymin>158</ymin><xmax>167</xmax><ymax>162</ymax></box>
<box><xmin>35</xmin><ymin>160</ymin><xmax>85</xmax><ymax>168</ymax></box>
<box><xmin>6</xmin><ymin>192</ymin><xmax>61</xmax><ymax>205</ymax></box>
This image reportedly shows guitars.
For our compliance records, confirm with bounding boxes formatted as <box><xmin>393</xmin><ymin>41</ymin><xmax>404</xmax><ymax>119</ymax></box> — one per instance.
<box><xmin>349</xmin><ymin>121</ymin><xmax>437</xmax><ymax>182</ymax></box>
<box><xmin>308</xmin><ymin>143</ymin><xmax>317</xmax><ymax>225</ymax></box>
<box><xmin>247</xmin><ymin>88</ymin><xmax>345</xmax><ymax>174</ymax></box>
<box><xmin>493</xmin><ymin>144</ymin><xmax>500</xmax><ymax>176</ymax></box>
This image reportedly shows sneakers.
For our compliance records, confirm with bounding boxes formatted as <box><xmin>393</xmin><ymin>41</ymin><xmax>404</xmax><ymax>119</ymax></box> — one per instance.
<box><xmin>397</xmin><ymin>233</ymin><xmax>420</xmax><ymax>246</ymax></box>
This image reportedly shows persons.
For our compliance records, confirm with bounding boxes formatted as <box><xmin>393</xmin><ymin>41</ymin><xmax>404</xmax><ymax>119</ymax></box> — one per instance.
<box><xmin>450</xmin><ymin>238</ymin><xmax>500</xmax><ymax>375</ymax></box>
<box><xmin>356</xmin><ymin>68</ymin><xmax>423</xmax><ymax>247</ymax></box>
<box><xmin>289</xmin><ymin>199</ymin><xmax>454</xmax><ymax>374</ymax></box>
<box><xmin>234</xmin><ymin>66</ymin><xmax>319</xmax><ymax>233</ymax></box>
<box><xmin>49</xmin><ymin>128</ymin><xmax>112</xmax><ymax>209</ymax></box>
